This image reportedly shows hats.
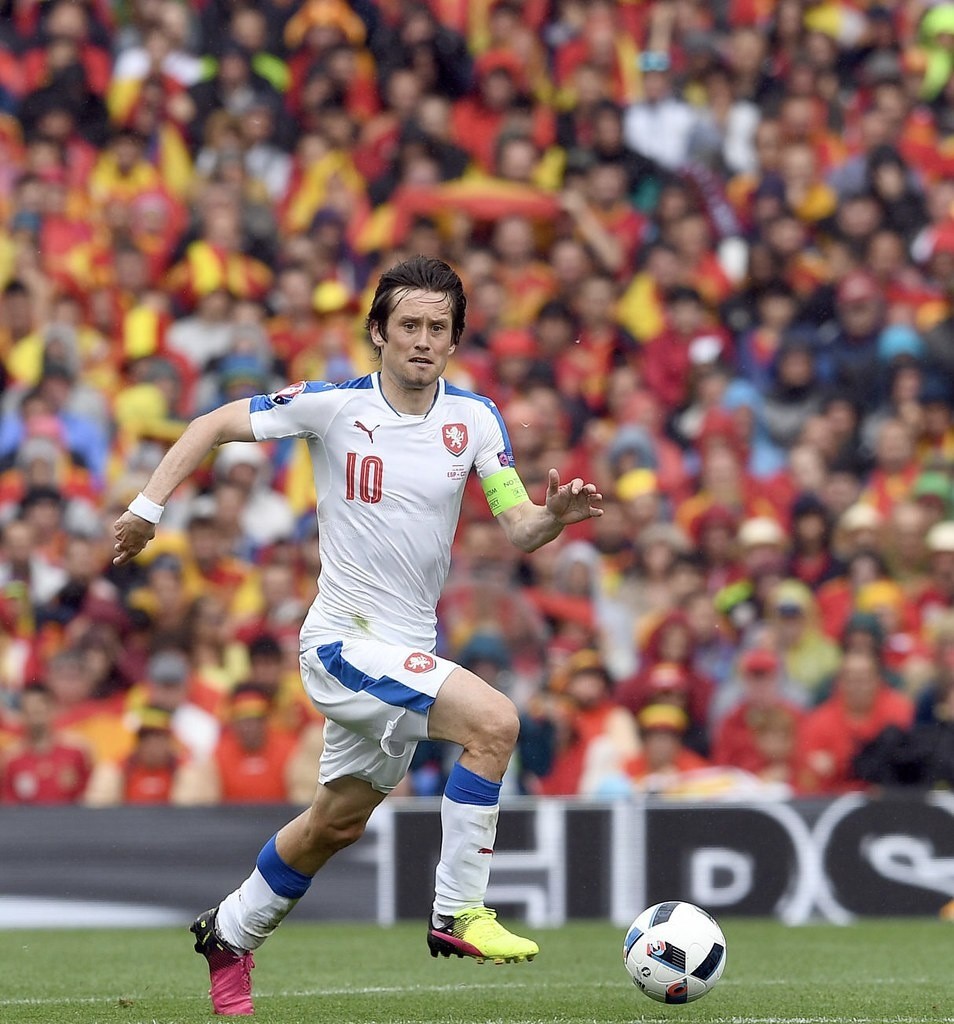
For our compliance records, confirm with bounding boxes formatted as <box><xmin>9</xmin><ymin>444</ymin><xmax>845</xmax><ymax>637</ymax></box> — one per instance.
<box><xmin>737</xmin><ymin>516</ymin><xmax>781</xmax><ymax>550</ymax></box>
<box><xmin>923</xmin><ymin>518</ymin><xmax>952</xmax><ymax>553</ymax></box>
<box><xmin>767</xmin><ymin>580</ymin><xmax>812</xmax><ymax>611</ymax></box>
<box><xmin>737</xmin><ymin>648</ymin><xmax>781</xmax><ymax>676</ymax></box>
<box><xmin>714</xmin><ymin>577</ymin><xmax>749</xmax><ymax>614</ymax></box>
<box><xmin>148</xmin><ymin>646</ymin><xmax>187</xmax><ymax>685</ymax></box>
<box><xmin>854</xmin><ymin>579</ymin><xmax>900</xmax><ymax>615</ymax></box>
<box><xmin>839</xmin><ymin>501</ymin><xmax>878</xmax><ymax>532</ymax></box>
<box><xmin>637</xmin><ymin>700</ymin><xmax>690</xmax><ymax>734</ymax></box>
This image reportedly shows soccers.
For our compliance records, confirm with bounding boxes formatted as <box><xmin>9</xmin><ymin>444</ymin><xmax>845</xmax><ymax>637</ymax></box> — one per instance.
<box><xmin>623</xmin><ymin>900</ymin><xmax>728</xmax><ymax>1004</ymax></box>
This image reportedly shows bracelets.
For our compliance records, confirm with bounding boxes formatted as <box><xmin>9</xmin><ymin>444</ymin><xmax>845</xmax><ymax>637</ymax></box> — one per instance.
<box><xmin>128</xmin><ymin>491</ymin><xmax>164</xmax><ymax>525</ymax></box>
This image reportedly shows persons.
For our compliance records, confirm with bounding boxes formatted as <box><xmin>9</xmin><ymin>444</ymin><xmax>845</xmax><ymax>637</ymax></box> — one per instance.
<box><xmin>0</xmin><ymin>0</ymin><xmax>954</xmax><ymax>801</ymax></box>
<box><xmin>110</xmin><ymin>254</ymin><xmax>605</xmax><ymax>1017</ymax></box>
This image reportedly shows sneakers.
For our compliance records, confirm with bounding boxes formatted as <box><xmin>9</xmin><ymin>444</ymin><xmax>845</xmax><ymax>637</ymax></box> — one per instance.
<box><xmin>189</xmin><ymin>906</ymin><xmax>256</xmax><ymax>1015</ymax></box>
<box><xmin>426</xmin><ymin>900</ymin><xmax>538</xmax><ymax>965</ymax></box>
